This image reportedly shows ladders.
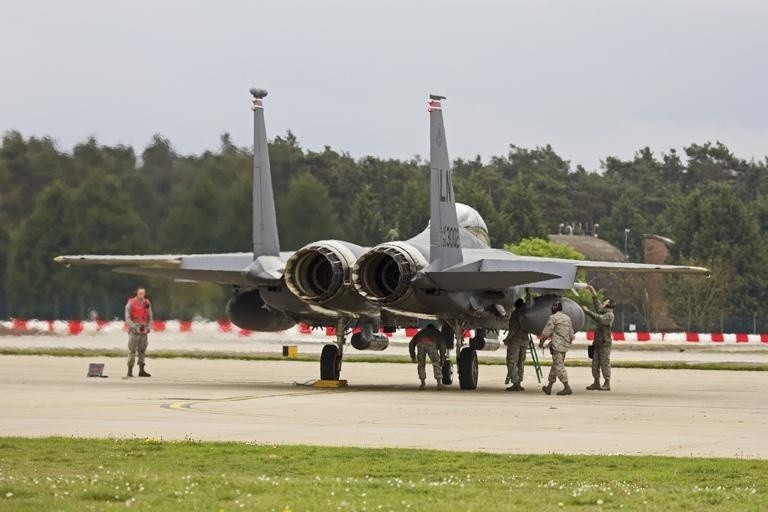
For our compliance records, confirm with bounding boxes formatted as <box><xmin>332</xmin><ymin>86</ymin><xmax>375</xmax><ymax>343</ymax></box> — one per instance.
<box><xmin>504</xmin><ymin>332</ymin><xmax>544</xmax><ymax>384</ymax></box>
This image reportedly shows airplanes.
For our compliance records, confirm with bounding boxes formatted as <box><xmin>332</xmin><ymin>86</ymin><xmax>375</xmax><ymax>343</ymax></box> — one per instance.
<box><xmin>53</xmin><ymin>87</ymin><xmax>711</xmax><ymax>390</ymax></box>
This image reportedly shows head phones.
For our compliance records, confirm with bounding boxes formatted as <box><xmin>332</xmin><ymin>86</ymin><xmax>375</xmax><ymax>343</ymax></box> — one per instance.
<box><xmin>605</xmin><ymin>303</ymin><xmax>616</xmax><ymax>308</ymax></box>
<box><xmin>552</xmin><ymin>300</ymin><xmax>559</xmax><ymax>312</ymax></box>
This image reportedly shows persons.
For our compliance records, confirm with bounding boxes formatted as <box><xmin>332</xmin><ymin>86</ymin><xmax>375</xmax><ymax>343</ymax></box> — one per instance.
<box><xmin>502</xmin><ymin>298</ymin><xmax>528</xmax><ymax>391</ymax></box>
<box><xmin>537</xmin><ymin>301</ymin><xmax>576</xmax><ymax>395</ymax></box>
<box><xmin>581</xmin><ymin>285</ymin><xmax>616</xmax><ymax>391</ymax></box>
<box><xmin>122</xmin><ymin>285</ymin><xmax>153</xmax><ymax>380</ymax></box>
<box><xmin>408</xmin><ymin>322</ymin><xmax>445</xmax><ymax>390</ymax></box>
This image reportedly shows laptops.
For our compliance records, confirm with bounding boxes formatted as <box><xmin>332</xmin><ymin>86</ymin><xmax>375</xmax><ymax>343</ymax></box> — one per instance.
<box><xmin>87</xmin><ymin>364</ymin><xmax>109</xmax><ymax>378</ymax></box>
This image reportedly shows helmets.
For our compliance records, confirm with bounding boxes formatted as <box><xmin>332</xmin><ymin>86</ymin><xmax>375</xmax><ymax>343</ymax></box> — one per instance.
<box><xmin>602</xmin><ymin>297</ymin><xmax>616</xmax><ymax>308</ymax></box>
<box><xmin>551</xmin><ymin>302</ymin><xmax>562</xmax><ymax>313</ymax></box>
<box><xmin>515</xmin><ymin>298</ymin><xmax>524</xmax><ymax>308</ymax></box>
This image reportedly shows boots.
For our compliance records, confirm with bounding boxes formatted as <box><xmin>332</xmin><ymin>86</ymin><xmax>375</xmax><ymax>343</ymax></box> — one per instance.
<box><xmin>586</xmin><ymin>379</ymin><xmax>601</xmax><ymax>390</ymax></box>
<box><xmin>138</xmin><ymin>365</ymin><xmax>151</xmax><ymax>377</ymax></box>
<box><xmin>598</xmin><ymin>379</ymin><xmax>611</xmax><ymax>391</ymax></box>
<box><xmin>556</xmin><ymin>382</ymin><xmax>574</xmax><ymax>395</ymax></box>
<box><xmin>419</xmin><ymin>378</ymin><xmax>443</xmax><ymax>389</ymax></box>
<box><xmin>127</xmin><ymin>365</ymin><xmax>133</xmax><ymax>377</ymax></box>
<box><xmin>542</xmin><ymin>382</ymin><xmax>554</xmax><ymax>395</ymax></box>
<box><xmin>505</xmin><ymin>383</ymin><xmax>526</xmax><ymax>392</ymax></box>
